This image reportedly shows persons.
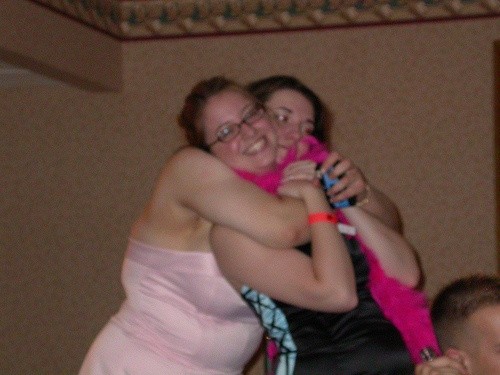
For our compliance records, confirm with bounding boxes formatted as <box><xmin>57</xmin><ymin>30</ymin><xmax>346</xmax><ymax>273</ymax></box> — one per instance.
<box><xmin>78</xmin><ymin>74</ymin><xmax>406</xmax><ymax>374</ymax></box>
<box><xmin>413</xmin><ymin>271</ymin><xmax>500</xmax><ymax>375</ymax></box>
<box><xmin>176</xmin><ymin>74</ymin><xmax>439</xmax><ymax>375</ymax></box>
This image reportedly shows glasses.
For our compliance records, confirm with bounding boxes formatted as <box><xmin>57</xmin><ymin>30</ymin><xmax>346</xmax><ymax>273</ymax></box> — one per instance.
<box><xmin>207</xmin><ymin>105</ymin><xmax>264</xmax><ymax>149</ymax></box>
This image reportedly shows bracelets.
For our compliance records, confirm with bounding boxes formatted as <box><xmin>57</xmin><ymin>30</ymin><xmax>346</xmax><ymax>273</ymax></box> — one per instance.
<box><xmin>307</xmin><ymin>211</ymin><xmax>357</xmax><ymax>237</ymax></box>
<box><xmin>356</xmin><ymin>182</ymin><xmax>372</xmax><ymax>208</ymax></box>
